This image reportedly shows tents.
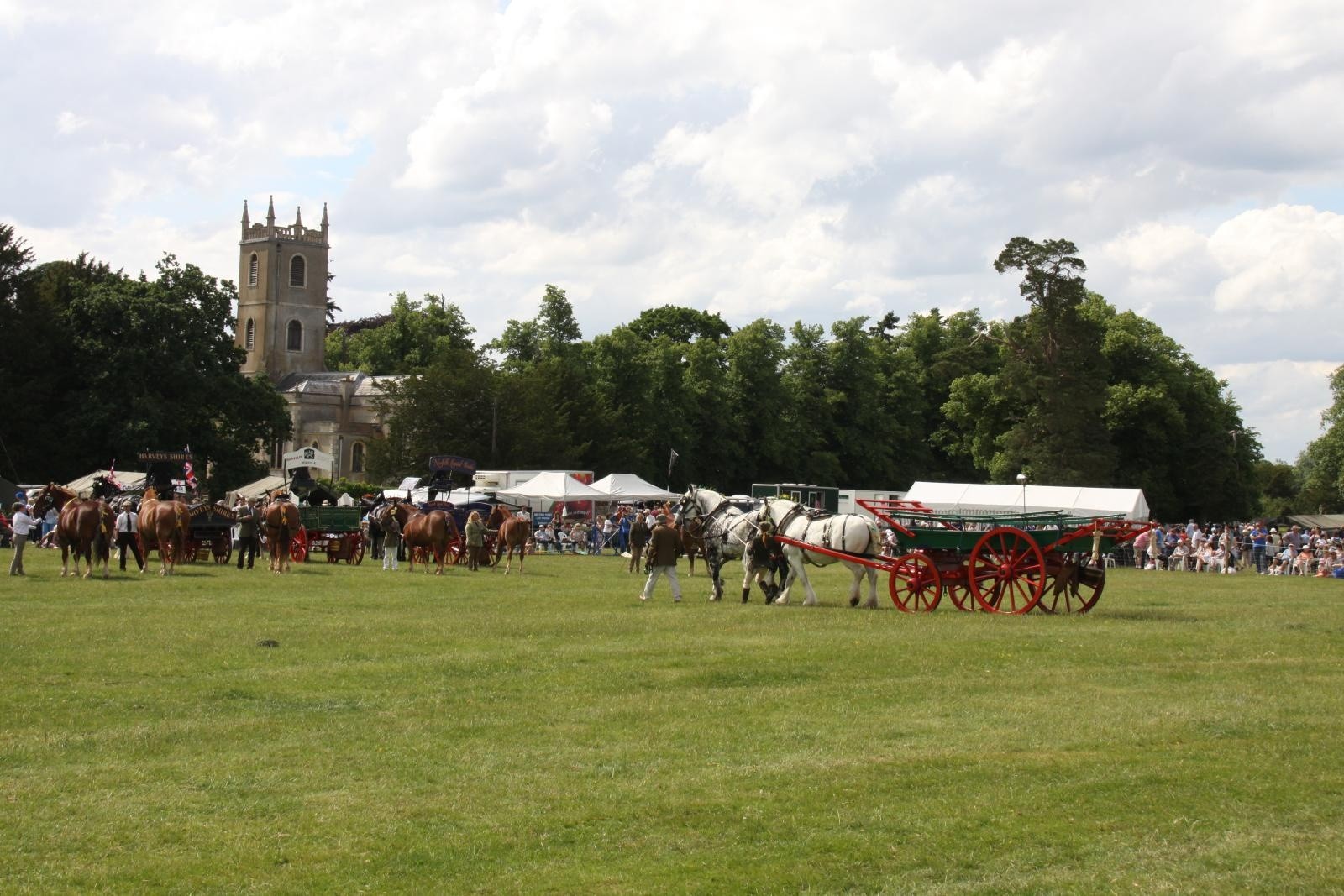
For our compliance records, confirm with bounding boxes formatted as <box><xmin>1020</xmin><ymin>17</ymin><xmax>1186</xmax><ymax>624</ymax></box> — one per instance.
<box><xmin>496</xmin><ymin>471</ymin><xmax>685</xmax><ymax>550</ymax></box>
<box><xmin>901</xmin><ymin>481</ymin><xmax>1150</xmax><ymax>528</ymax></box>
<box><xmin>61</xmin><ymin>470</ymin><xmax>148</xmax><ymax>499</ymax></box>
<box><xmin>226</xmin><ymin>476</ymin><xmax>292</xmax><ymax>511</ymax></box>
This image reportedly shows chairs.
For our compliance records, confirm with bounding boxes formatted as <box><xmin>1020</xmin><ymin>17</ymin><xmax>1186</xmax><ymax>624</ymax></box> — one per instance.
<box><xmin>1155</xmin><ymin>553</ymin><xmax>1231</xmax><ymax>572</ymax></box>
<box><xmin>1271</xmin><ymin>556</ymin><xmax>1315</xmax><ymax>576</ymax></box>
<box><xmin>558</xmin><ymin>532</ymin><xmax>571</xmax><ymax>551</ymax></box>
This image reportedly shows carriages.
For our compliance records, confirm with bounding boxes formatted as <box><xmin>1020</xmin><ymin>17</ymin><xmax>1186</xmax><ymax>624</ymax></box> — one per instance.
<box><xmin>674</xmin><ymin>472</ymin><xmax>1163</xmax><ymax>616</ymax></box>
<box><xmin>362</xmin><ymin>484</ymin><xmax>507</xmax><ymax>569</ymax></box>
<box><xmin>260</xmin><ymin>496</ymin><xmax>365</xmax><ymax>567</ymax></box>
<box><xmin>29</xmin><ymin>468</ymin><xmax>240</xmax><ymax>567</ymax></box>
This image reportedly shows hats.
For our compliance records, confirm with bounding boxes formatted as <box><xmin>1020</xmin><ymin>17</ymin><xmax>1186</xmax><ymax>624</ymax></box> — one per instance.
<box><xmin>655</xmin><ymin>514</ymin><xmax>666</xmax><ymax>524</ymax></box>
<box><xmin>16</xmin><ymin>492</ymin><xmax>23</xmax><ymax>497</ymax></box>
<box><xmin>123</xmin><ymin>500</ymin><xmax>131</xmax><ymax>506</ymax></box>
<box><xmin>1269</xmin><ymin>528</ymin><xmax>1277</xmax><ymax>532</ymax></box>
<box><xmin>1177</xmin><ymin>539</ymin><xmax>1182</xmax><ymax>543</ymax></box>
<box><xmin>1319</xmin><ymin>558</ymin><xmax>1325</xmax><ymax>563</ymax></box>
<box><xmin>539</xmin><ymin>524</ymin><xmax>544</xmax><ymax>527</ymax></box>
<box><xmin>760</xmin><ymin>522</ymin><xmax>771</xmax><ymax>531</ymax></box>
<box><xmin>1303</xmin><ymin>545</ymin><xmax>1309</xmax><ymax>548</ymax></box>
<box><xmin>1292</xmin><ymin>525</ymin><xmax>1301</xmax><ymax>530</ymax></box>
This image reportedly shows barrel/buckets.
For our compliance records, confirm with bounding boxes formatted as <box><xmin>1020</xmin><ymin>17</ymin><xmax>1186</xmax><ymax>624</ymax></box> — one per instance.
<box><xmin>1084</xmin><ymin>557</ymin><xmax>1104</xmax><ymax>585</ymax></box>
<box><xmin>330</xmin><ymin>534</ymin><xmax>340</xmax><ymax>551</ymax></box>
<box><xmin>195</xmin><ymin>541</ymin><xmax>210</xmax><ymax>561</ymax></box>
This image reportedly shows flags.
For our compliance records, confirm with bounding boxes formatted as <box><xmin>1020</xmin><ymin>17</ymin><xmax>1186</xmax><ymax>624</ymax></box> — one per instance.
<box><xmin>110</xmin><ymin>472</ymin><xmax>121</xmax><ymax>488</ymax></box>
<box><xmin>181</xmin><ymin>444</ymin><xmax>200</xmax><ymax>489</ymax></box>
<box><xmin>667</xmin><ymin>450</ymin><xmax>679</xmax><ymax>486</ymax></box>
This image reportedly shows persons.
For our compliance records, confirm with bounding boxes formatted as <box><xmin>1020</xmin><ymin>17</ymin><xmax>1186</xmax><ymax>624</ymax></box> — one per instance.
<box><xmin>629</xmin><ymin>513</ymin><xmax>652</xmax><ymax>573</ymax></box>
<box><xmin>115</xmin><ymin>501</ymin><xmax>144</xmax><ymax>572</ymax></box>
<box><xmin>0</xmin><ymin>491</ymin><xmax>58</xmax><ymax>549</ymax></box>
<box><xmin>1133</xmin><ymin>516</ymin><xmax>1344</xmax><ymax>579</ymax></box>
<box><xmin>382</xmin><ymin>510</ymin><xmax>401</xmax><ymax>571</ymax></box>
<box><xmin>741</xmin><ymin>521</ymin><xmax>776</xmax><ymax>605</ymax></box>
<box><xmin>639</xmin><ymin>513</ymin><xmax>682</xmax><ymax>603</ymax></box>
<box><xmin>465</xmin><ymin>511</ymin><xmax>499</xmax><ymax>571</ymax></box>
<box><xmin>9</xmin><ymin>502</ymin><xmax>42</xmax><ymax>576</ymax></box>
<box><xmin>368</xmin><ymin>517</ymin><xmax>386</xmax><ymax>560</ymax></box>
<box><xmin>876</xmin><ymin>509</ymin><xmax>1059</xmax><ymax>557</ymax></box>
<box><xmin>232</xmin><ymin>496</ymin><xmax>264</xmax><ymax>570</ymax></box>
<box><xmin>517</xmin><ymin>504</ymin><xmax>661</xmax><ymax>555</ymax></box>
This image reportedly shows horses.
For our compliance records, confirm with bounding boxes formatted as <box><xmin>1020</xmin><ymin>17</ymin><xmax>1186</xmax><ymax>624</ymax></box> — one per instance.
<box><xmin>676</xmin><ymin>484</ymin><xmax>879</xmax><ymax>606</ymax></box>
<box><xmin>33</xmin><ymin>481</ymin><xmax>530</xmax><ymax>578</ymax></box>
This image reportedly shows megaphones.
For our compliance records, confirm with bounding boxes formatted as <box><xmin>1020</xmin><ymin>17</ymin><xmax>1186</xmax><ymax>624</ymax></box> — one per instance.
<box><xmin>1017</xmin><ymin>473</ymin><xmax>1027</xmax><ymax>483</ymax></box>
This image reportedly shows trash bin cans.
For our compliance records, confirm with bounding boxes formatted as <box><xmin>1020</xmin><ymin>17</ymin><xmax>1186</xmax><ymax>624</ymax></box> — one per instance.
<box><xmin>40</xmin><ymin>520</ymin><xmax>54</xmax><ymax>539</ymax></box>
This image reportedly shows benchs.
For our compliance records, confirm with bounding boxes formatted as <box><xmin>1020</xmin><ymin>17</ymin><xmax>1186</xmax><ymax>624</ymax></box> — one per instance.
<box><xmin>884</xmin><ymin>510</ymin><xmax>1125</xmax><ymax>552</ymax></box>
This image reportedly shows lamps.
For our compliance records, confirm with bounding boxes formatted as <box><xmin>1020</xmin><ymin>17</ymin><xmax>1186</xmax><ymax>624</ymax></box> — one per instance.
<box><xmin>1017</xmin><ymin>474</ymin><xmax>1027</xmax><ymax>513</ymax></box>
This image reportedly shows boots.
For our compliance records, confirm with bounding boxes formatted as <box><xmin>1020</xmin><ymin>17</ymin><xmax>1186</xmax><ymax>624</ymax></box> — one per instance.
<box><xmin>759</xmin><ymin>581</ymin><xmax>774</xmax><ymax>604</ymax></box>
<box><xmin>742</xmin><ymin>587</ymin><xmax>750</xmax><ymax>603</ymax></box>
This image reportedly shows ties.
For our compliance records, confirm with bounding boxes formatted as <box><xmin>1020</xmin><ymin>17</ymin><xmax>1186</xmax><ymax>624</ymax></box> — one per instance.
<box><xmin>127</xmin><ymin>514</ymin><xmax>131</xmax><ymax>532</ymax></box>
<box><xmin>640</xmin><ymin>523</ymin><xmax>642</xmax><ymax>531</ymax></box>
<box><xmin>249</xmin><ymin>507</ymin><xmax>252</xmax><ymax>515</ymax></box>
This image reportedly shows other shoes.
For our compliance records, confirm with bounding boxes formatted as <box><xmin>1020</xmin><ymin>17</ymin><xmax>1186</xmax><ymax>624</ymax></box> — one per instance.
<box><xmin>1275</xmin><ymin>571</ymin><xmax>1281</xmax><ymax>575</ymax></box>
<box><xmin>1269</xmin><ymin>569</ymin><xmax>1274</xmax><ymax>576</ymax></box>
<box><xmin>1135</xmin><ymin>565</ymin><xmax>1138</xmax><ymax>568</ymax></box>
<box><xmin>1138</xmin><ymin>566</ymin><xmax>1143</xmax><ymax>568</ymax></box>
<box><xmin>640</xmin><ymin>595</ymin><xmax>651</xmax><ymax>600</ymax></box>
<box><xmin>674</xmin><ymin>597</ymin><xmax>680</xmax><ymax>602</ymax></box>
